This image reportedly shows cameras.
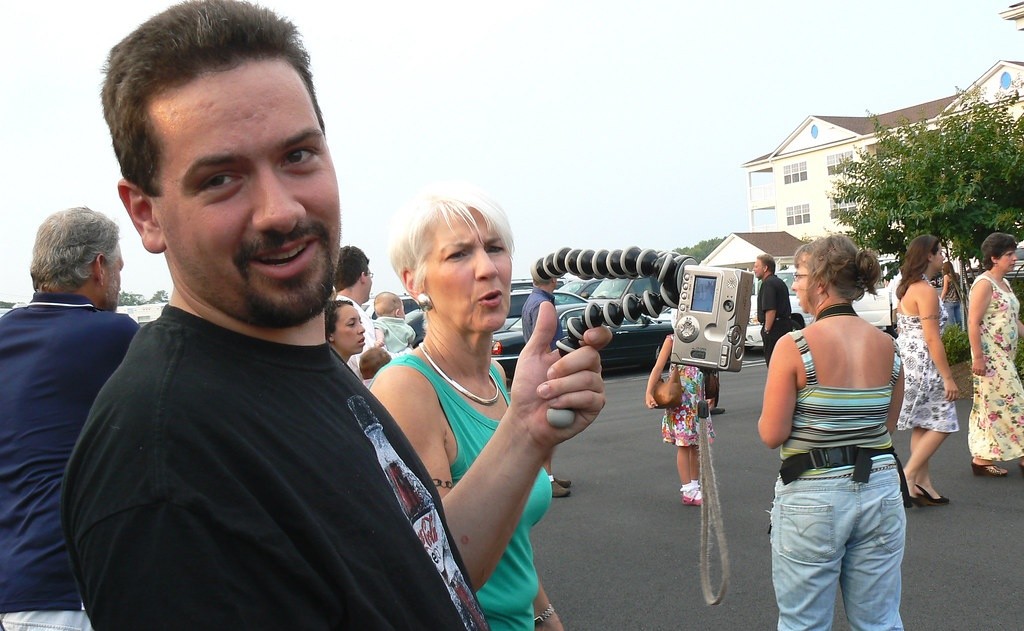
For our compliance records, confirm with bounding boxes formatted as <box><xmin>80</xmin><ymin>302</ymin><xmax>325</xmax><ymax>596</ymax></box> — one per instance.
<box><xmin>670</xmin><ymin>265</ymin><xmax>755</xmax><ymax>372</ymax></box>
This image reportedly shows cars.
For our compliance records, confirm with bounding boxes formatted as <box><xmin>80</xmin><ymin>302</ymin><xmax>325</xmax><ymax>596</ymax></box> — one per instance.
<box><xmin>362</xmin><ymin>237</ymin><xmax>1024</xmax><ymax>385</ymax></box>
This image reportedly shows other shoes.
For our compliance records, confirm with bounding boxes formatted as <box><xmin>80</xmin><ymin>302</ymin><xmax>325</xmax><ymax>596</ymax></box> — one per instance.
<box><xmin>710</xmin><ymin>408</ymin><xmax>725</xmax><ymax>415</ymax></box>
<box><xmin>554</xmin><ymin>478</ymin><xmax>571</xmax><ymax>487</ymax></box>
<box><xmin>551</xmin><ymin>480</ymin><xmax>570</xmax><ymax>496</ymax></box>
<box><xmin>682</xmin><ymin>491</ymin><xmax>702</xmax><ymax>505</ymax></box>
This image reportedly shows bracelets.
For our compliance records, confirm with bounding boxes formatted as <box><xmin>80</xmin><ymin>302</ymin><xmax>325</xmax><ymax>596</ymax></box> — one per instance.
<box><xmin>764</xmin><ymin>330</ymin><xmax>769</xmax><ymax>332</ymax></box>
<box><xmin>535</xmin><ymin>604</ymin><xmax>555</xmax><ymax>623</ymax></box>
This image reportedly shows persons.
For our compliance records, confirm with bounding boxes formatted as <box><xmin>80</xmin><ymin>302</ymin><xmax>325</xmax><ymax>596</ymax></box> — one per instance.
<box><xmin>0</xmin><ymin>206</ymin><xmax>140</xmax><ymax>631</ymax></box>
<box><xmin>895</xmin><ymin>235</ymin><xmax>959</xmax><ymax>506</ymax></box>
<box><xmin>645</xmin><ymin>334</ymin><xmax>714</xmax><ymax>505</ymax></box>
<box><xmin>967</xmin><ymin>232</ymin><xmax>1024</xmax><ymax>476</ymax></box>
<box><xmin>368</xmin><ymin>187</ymin><xmax>564</xmax><ymax>631</ymax></box>
<box><xmin>57</xmin><ymin>0</ymin><xmax>612</xmax><ymax>631</ymax></box>
<box><xmin>941</xmin><ymin>261</ymin><xmax>961</xmax><ymax>328</ymax></box>
<box><xmin>521</xmin><ymin>278</ymin><xmax>570</xmax><ymax>496</ymax></box>
<box><xmin>757</xmin><ymin>236</ymin><xmax>907</xmax><ymax>631</ymax></box>
<box><xmin>705</xmin><ymin>369</ymin><xmax>725</xmax><ymax>414</ymax></box>
<box><xmin>754</xmin><ymin>255</ymin><xmax>792</xmax><ymax>368</ymax></box>
<box><xmin>327</xmin><ymin>245</ymin><xmax>417</xmax><ymax>389</ymax></box>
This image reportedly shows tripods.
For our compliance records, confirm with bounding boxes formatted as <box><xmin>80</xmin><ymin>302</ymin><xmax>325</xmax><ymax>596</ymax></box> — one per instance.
<box><xmin>529</xmin><ymin>247</ymin><xmax>698</xmax><ymax>428</ymax></box>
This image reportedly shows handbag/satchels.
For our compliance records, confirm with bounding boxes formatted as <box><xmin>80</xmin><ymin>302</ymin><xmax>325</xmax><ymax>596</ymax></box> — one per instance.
<box><xmin>653</xmin><ymin>367</ymin><xmax>683</xmax><ymax>409</ymax></box>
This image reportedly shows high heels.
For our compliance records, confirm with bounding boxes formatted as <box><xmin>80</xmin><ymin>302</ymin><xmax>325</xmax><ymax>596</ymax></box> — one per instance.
<box><xmin>914</xmin><ymin>484</ymin><xmax>949</xmax><ymax>505</ymax></box>
<box><xmin>971</xmin><ymin>461</ymin><xmax>1007</xmax><ymax>476</ymax></box>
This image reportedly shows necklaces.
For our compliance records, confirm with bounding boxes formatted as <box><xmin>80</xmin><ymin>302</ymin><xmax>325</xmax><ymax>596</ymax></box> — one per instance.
<box><xmin>419</xmin><ymin>343</ymin><xmax>499</xmax><ymax>405</ymax></box>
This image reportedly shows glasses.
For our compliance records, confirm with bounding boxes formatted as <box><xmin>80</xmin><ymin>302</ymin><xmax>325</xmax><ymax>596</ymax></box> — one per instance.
<box><xmin>364</xmin><ymin>273</ymin><xmax>373</xmax><ymax>277</ymax></box>
<box><xmin>793</xmin><ymin>273</ymin><xmax>811</xmax><ymax>280</ymax></box>
